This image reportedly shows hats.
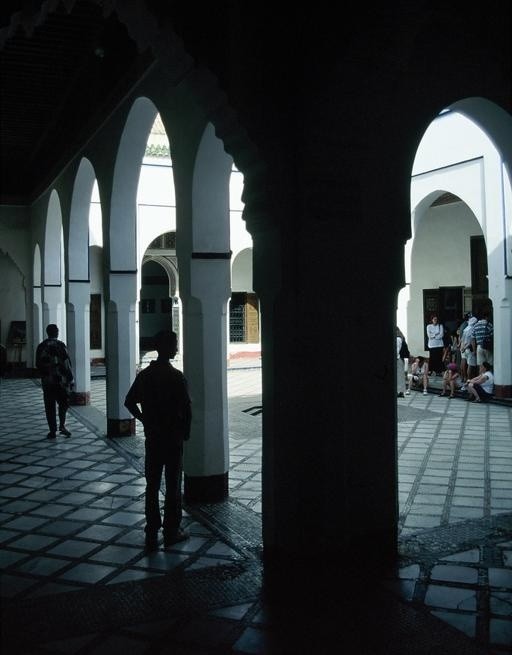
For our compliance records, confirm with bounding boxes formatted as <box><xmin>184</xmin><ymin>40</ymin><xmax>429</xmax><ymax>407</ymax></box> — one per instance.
<box><xmin>447</xmin><ymin>363</ymin><xmax>457</xmax><ymax>371</ymax></box>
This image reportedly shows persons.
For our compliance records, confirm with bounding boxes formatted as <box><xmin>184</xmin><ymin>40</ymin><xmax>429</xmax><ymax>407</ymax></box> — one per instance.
<box><xmin>36</xmin><ymin>323</ymin><xmax>73</xmax><ymax>438</ymax></box>
<box><xmin>124</xmin><ymin>328</ymin><xmax>193</xmax><ymax>546</ymax></box>
<box><xmin>397</xmin><ymin>313</ymin><xmax>494</xmax><ymax>403</ymax></box>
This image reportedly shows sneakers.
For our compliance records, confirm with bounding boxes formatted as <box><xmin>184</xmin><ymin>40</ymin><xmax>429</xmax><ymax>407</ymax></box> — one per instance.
<box><xmin>145</xmin><ymin>530</ymin><xmax>158</xmax><ymax>545</ymax></box>
<box><xmin>397</xmin><ymin>388</ymin><xmax>483</xmax><ymax>404</ymax></box>
<box><xmin>164</xmin><ymin>528</ymin><xmax>189</xmax><ymax>547</ymax></box>
<box><xmin>48</xmin><ymin>431</ymin><xmax>56</xmax><ymax>438</ymax></box>
<box><xmin>59</xmin><ymin>428</ymin><xmax>71</xmax><ymax>436</ymax></box>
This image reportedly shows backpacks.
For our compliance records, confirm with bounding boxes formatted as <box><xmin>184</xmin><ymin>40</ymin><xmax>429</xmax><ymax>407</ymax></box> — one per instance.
<box><xmin>400</xmin><ymin>340</ymin><xmax>409</xmax><ymax>358</ymax></box>
<box><xmin>48</xmin><ymin>342</ymin><xmax>73</xmax><ymax>386</ymax></box>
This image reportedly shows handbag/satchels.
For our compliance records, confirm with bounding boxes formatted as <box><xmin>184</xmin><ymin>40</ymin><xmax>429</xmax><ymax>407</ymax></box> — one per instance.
<box><xmin>482</xmin><ymin>339</ymin><xmax>492</xmax><ymax>352</ymax></box>
